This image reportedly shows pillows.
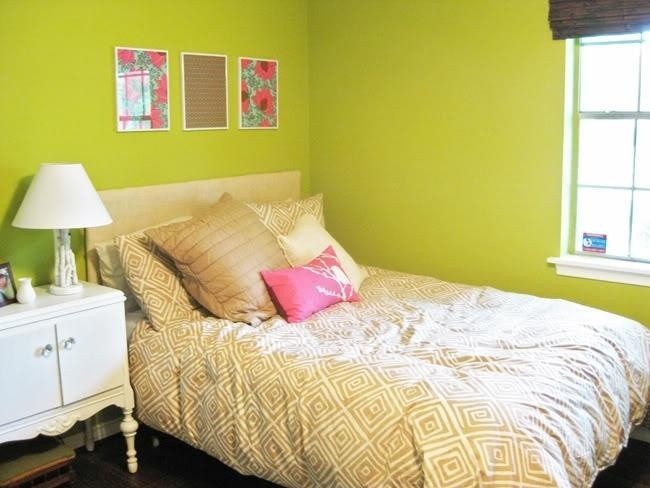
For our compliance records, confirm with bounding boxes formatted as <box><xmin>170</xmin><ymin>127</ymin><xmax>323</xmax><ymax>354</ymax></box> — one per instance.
<box><xmin>141</xmin><ymin>191</ymin><xmax>294</xmax><ymax>328</ymax></box>
<box><xmin>95</xmin><ymin>239</ymin><xmax>138</xmax><ymax>314</ymax></box>
<box><xmin>115</xmin><ymin>232</ymin><xmax>212</xmax><ymax>331</ymax></box>
<box><xmin>276</xmin><ymin>213</ymin><xmax>369</xmax><ymax>298</ymax></box>
<box><xmin>258</xmin><ymin>243</ymin><xmax>365</xmax><ymax>324</ymax></box>
<box><xmin>241</xmin><ymin>192</ymin><xmax>327</xmax><ymax>241</ymax></box>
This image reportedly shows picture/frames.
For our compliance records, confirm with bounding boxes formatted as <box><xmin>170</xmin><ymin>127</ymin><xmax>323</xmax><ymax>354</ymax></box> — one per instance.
<box><xmin>0</xmin><ymin>262</ymin><xmax>17</xmax><ymax>308</ymax></box>
<box><xmin>114</xmin><ymin>43</ymin><xmax>280</xmax><ymax>134</ymax></box>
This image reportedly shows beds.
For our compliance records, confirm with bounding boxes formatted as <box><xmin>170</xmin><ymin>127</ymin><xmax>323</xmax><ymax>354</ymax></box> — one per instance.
<box><xmin>86</xmin><ymin>167</ymin><xmax>650</xmax><ymax>488</ymax></box>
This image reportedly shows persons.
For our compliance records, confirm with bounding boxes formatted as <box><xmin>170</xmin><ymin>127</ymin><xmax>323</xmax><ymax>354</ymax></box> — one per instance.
<box><xmin>0</xmin><ymin>266</ymin><xmax>15</xmax><ymax>306</ymax></box>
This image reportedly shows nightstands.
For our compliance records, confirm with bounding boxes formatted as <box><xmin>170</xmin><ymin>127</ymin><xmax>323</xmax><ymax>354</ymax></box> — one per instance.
<box><xmin>0</xmin><ymin>279</ymin><xmax>141</xmax><ymax>476</ymax></box>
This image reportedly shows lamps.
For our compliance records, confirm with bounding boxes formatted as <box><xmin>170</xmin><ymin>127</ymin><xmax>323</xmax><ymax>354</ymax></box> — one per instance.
<box><xmin>11</xmin><ymin>161</ymin><xmax>114</xmax><ymax>296</ymax></box>
